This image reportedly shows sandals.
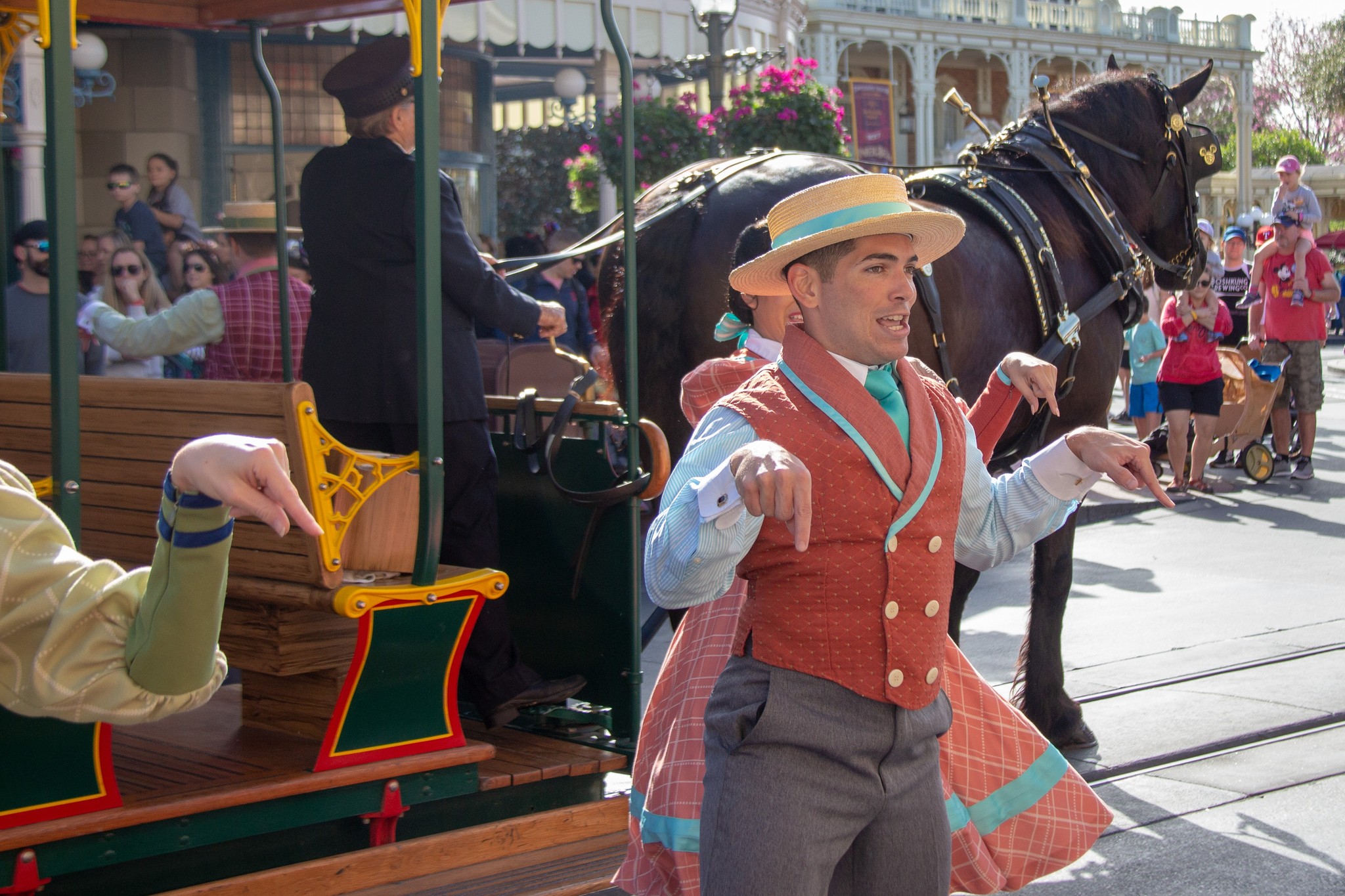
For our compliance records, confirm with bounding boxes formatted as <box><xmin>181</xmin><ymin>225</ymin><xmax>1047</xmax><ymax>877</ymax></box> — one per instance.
<box><xmin>1189</xmin><ymin>478</ymin><xmax>1213</xmax><ymax>494</ymax></box>
<box><xmin>1166</xmin><ymin>480</ymin><xmax>1185</xmax><ymax>493</ymax></box>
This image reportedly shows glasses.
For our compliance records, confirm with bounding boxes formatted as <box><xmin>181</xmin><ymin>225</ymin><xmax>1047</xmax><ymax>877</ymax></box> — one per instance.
<box><xmin>113</xmin><ymin>265</ymin><xmax>143</xmax><ymax>277</ymax></box>
<box><xmin>18</xmin><ymin>242</ymin><xmax>50</xmax><ymax>251</ymax></box>
<box><xmin>1197</xmin><ymin>276</ymin><xmax>1212</xmax><ymax>288</ymax></box>
<box><xmin>182</xmin><ymin>263</ymin><xmax>210</xmax><ymax>273</ymax></box>
<box><xmin>569</xmin><ymin>257</ymin><xmax>587</xmax><ymax>264</ymax></box>
<box><xmin>107</xmin><ymin>182</ymin><xmax>134</xmax><ymax>190</ymax></box>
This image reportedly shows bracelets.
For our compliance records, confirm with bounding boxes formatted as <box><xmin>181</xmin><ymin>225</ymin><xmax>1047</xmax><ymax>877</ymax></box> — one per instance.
<box><xmin>1190</xmin><ymin>310</ymin><xmax>1197</xmax><ymax>322</ymax></box>
<box><xmin>1308</xmin><ymin>288</ymin><xmax>1314</xmax><ymax>301</ymax></box>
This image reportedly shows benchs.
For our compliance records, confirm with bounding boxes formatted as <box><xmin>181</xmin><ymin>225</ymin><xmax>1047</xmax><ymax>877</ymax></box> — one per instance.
<box><xmin>0</xmin><ymin>370</ymin><xmax>508</xmax><ymax>774</ymax></box>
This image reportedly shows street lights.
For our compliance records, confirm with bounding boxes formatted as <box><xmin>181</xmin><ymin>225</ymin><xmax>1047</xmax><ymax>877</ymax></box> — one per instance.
<box><xmin>687</xmin><ymin>2</ymin><xmax>739</xmax><ymax>137</ymax></box>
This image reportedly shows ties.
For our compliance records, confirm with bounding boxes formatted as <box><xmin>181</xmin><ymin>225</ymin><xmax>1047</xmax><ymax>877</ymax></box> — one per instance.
<box><xmin>869</xmin><ymin>364</ymin><xmax>911</xmax><ymax>454</ymax></box>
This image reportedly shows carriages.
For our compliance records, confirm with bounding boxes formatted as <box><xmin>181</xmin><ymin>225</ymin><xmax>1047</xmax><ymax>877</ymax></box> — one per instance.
<box><xmin>3</xmin><ymin>1</ymin><xmax>1231</xmax><ymax>896</ymax></box>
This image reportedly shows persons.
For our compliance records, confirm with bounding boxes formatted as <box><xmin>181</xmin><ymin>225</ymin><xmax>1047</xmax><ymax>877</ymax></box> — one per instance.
<box><xmin>609</xmin><ymin>217</ymin><xmax>1112</xmax><ymax>896</ymax></box>
<box><xmin>75</xmin><ymin>201</ymin><xmax>308</xmax><ymax>385</ymax></box>
<box><xmin>0</xmin><ymin>152</ymin><xmax>311</xmax><ymax>378</ymax></box>
<box><xmin>0</xmin><ymin>431</ymin><xmax>324</xmax><ymax>726</ymax></box>
<box><xmin>464</xmin><ymin>229</ymin><xmax>602</xmax><ymax>367</ymax></box>
<box><xmin>652</xmin><ymin>172</ymin><xmax>1175</xmax><ymax>896</ymax></box>
<box><xmin>1121</xmin><ymin>156</ymin><xmax>1340</xmax><ymax>488</ymax></box>
<box><xmin>299</xmin><ymin>34</ymin><xmax>612</xmax><ymax>738</ymax></box>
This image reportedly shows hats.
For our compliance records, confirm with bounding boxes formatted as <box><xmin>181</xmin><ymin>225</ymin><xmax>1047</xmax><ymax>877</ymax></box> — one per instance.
<box><xmin>16</xmin><ymin>221</ymin><xmax>51</xmax><ymax>239</ymax></box>
<box><xmin>1197</xmin><ymin>219</ymin><xmax>1214</xmax><ymax>237</ymax></box>
<box><xmin>1255</xmin><ymin>226</ymin><xmax>1275</xmax><ymax>246</ymax></box>
<box><xmin>322</xmin><ymin>35</ymin><xmax>417</xmax><ymax>119</ymax></box>
<box><xmin>1272</xmin><ymin>156</ymin><xmax>1300</xmax><ymax>175</ymax></box>
<box><xmin>1270</xmin><ymin>212</ymin><xmax>1300</xmax><ymax>227</ymax></box>
<box><xmin>1223</xmin><ymin>226</ymin><xmax>1247</xmax><ymax>246</ymax></box>
<box><xmin>200</xmin><ymin>202</ymin><xmax>304</xmax><ymax>234</ymax></box>
<box><xmin>728</xmin><ymin>174</ymin><xmax>965</xmax><ymax>297</ymax></box>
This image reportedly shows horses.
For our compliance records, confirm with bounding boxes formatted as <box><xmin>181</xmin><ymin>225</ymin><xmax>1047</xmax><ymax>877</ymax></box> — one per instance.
<box><xmin>585</xmin><ymin>44</ymin><xmax>1225</xmax><ymax>756</ymax></box>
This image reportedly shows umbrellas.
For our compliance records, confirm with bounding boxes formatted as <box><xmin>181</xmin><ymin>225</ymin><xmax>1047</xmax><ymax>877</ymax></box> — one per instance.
<box><xmin>1314</xmin><ymin>231</ymin><xmax>1345</xmax><ymax>249</ymax></box>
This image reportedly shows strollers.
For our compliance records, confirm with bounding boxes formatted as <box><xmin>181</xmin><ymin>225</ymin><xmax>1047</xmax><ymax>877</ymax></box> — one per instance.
<box><xmin>1140</xmin><ymin>337</ymin><xmax>1291</xmax><ymax>483</ymax></box>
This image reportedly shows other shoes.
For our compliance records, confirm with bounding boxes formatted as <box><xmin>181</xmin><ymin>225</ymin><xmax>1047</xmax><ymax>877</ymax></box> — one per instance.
<box><xmin>486</xmin><ymin>671</ymin><xmax>587</xmax><ymax>730</ymax></box>
<box><xmin>1208</xmin><ymin>330</ymin><xmax>1224</xmax><ymax>343</ymax></box>
<box><xmin>1172</xmin><ymin>332</ymin><xmax>1188</xmax><ymax>342</ymax></box>
<box><xmin>1110</xmin><ymin>412</ymin><xmax>1134</xmax><ymax>425</ymax></box>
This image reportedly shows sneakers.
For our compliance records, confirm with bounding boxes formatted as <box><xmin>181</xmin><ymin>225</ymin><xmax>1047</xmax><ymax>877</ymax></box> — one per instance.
<box><xmin>1210</xmin><ymin>452</ymin><xmax>1236</xmax><ymax>468</ymax></box>
<box><xmin>1271</xmin><ymin>458</ymin><xmax>1292</xmax><ymax>477</ymax></box>
<box><xmin>1291</xmin><ymin>291</ymin><xmax>1304</xmax><ymax>307</ymax></box>
<box><xmin>1291</xmin><ymin>459</ymin><xmax>1314</xmax><ymax>480</ymax></box>
<box><xmin>1236</xmin><ymin>291</ymin><xmax>1262</xmax><ymax>310</ymax></box>
